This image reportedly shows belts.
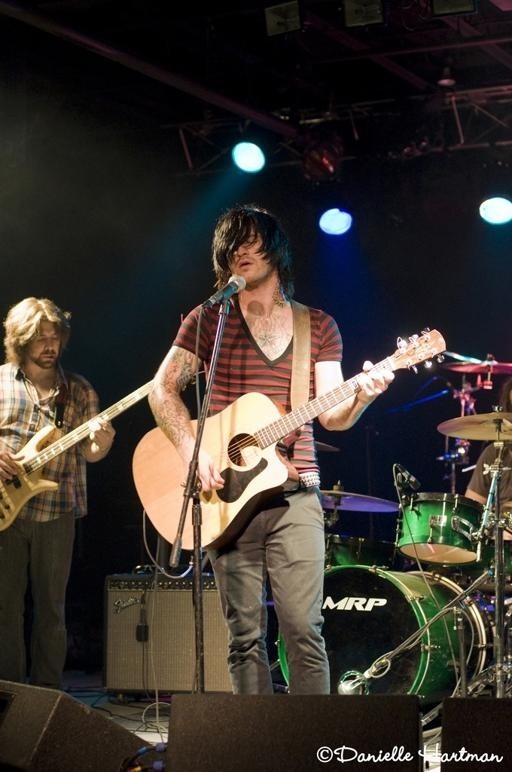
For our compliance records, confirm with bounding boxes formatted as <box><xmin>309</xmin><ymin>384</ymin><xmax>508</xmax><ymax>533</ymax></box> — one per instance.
<box><xmin>298</xmin><ymin>471</ymin><xmax>323</xmax><ymax>490</ymax></box>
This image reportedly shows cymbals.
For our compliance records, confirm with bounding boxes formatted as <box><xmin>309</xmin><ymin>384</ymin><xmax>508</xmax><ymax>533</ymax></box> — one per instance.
<box><xmin>444</xmin><ymin>360</ymin><xmax>512</xmax><ymax>375</ymax></box>
<box><xmin>320</xmin><ymin>490</ymin><xmax>401</xmax><ymax>514</ymax></box>
<box><xmin>437</xmin><ymin>413</ymin><xmax>512</xmax><ymax>441</ymax></box>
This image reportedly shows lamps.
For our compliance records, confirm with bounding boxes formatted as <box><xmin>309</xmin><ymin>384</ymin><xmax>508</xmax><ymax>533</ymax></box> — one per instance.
<box><xmin>316</xmin><ymin>185</ymin><xmax>353</xmax><ymax>237</ymax></box>
<box><xmin>471</xmin><ymin>173</ymin><xmax>512</xmax><ymax>225</ymax></box>
<box><xmin>228</xmin><ymin>122</ymin><xmax>279</xmax><ymax>177</ymax></box>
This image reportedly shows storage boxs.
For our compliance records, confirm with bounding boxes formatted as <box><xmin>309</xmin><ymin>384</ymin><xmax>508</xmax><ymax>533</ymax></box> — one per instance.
<box><xmin>104</xmin><ymin>572</ymin><xmax>235</xmax><ymax>702</ymax></box>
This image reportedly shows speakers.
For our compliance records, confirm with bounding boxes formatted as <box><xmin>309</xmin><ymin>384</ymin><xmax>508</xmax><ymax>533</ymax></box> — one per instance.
<box><xmin>0</xmin><ymin>679</ymin><xmax>165</xmax><ymax>772</ymax></box>
<box><xmin>164</xmin><ymin>694</ymin><xmax>424</xmax><ymax>772</ymax></box>
<box><xmin>441</xmin><ymin>697</ymin><xmax>512</xmax><ymax>772</ymax></box>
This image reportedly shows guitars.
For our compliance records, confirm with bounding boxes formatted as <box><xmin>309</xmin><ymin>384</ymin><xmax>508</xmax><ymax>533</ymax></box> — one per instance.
<box><xmin>132</xmin><ymin>325</ymin><xmax>447</xmax><ymax>552</ymax></box>
<box><xmin>0</xmin><ymin>373</ymin><xmax>196</xmax><ymax>532</ymax></box>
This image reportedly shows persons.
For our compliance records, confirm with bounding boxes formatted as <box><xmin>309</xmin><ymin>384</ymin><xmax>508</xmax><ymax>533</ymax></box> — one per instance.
<box><xmin>0</xmin><ymin>293</ymin><xmax>117</xmax><ymax>687</ymax></box>
<box><xmin>145</xmin><ymin>201</ymin><xmax>398</xmax><ymax>695</ymax></box>
<box><xmin>464</xmin><ymin>379</ymin><xmax>512</xmax><ymax>541</ymax></box>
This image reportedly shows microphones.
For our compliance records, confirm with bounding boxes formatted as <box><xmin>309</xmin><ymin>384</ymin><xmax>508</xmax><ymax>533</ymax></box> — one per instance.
<box><xmin>202</xmin><ymin>275</ymin><xmax>246</xmax><ymax>308</ymax></box>
<box><xmin>397</xmin><ymin>464</ymin><xmax>422</xmax><ymax>490</ymax></box>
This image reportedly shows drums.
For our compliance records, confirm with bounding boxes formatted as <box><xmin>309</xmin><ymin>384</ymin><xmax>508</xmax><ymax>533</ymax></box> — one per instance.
<box><xmin>426</xmin><ymin>538</ymin><xmax>512</xmax><ymax>599</ymax></box>
<box><xmin>275</xmin><ymin>564</ymin><xmax>492</xmax><ymax>731</ymax></box>
<box><xmin>394</xmin><ymin>491</ymin><xmax>484</xmax><ymax>567</ymax></box>
<box><xmin>326</xmin><ymin>534</ymin><xmax>404</xmax><ymax>571</ymax></box>
